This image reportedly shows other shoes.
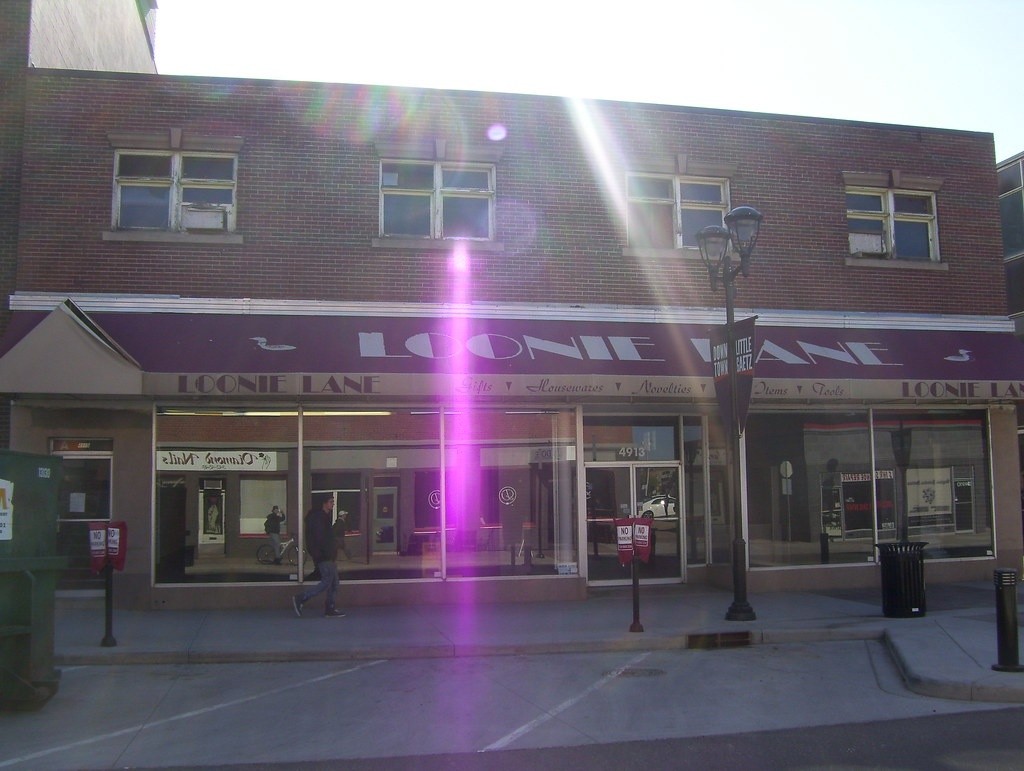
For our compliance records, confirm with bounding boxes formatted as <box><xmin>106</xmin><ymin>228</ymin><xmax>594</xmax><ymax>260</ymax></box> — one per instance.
<box><xmin>274</xmin><ymin>558</ymin><xmax>281</xmax><ymax>565</ymax></box>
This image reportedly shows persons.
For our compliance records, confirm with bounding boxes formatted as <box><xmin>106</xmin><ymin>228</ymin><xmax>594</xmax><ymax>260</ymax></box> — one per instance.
<box><xmin>332</xmin><ymin>511</ymin><xmax>354</xmax><ymax>562</ymax></box>
<box><xmin>265</xmin><ymin>506</ymin><xmax>286</xmax><ymax>566</ymax></box>
<box><xmin>292</xmin><ymin>493</ymin><xmax>346</xmax><ymax>619</ymax></box>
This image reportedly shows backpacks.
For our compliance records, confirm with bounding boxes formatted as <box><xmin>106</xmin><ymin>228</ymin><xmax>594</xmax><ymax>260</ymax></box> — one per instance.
<box><xmin>264</xmin><ymin>514</ymin><xmax>272</xmax><ymax>534</ymax></box>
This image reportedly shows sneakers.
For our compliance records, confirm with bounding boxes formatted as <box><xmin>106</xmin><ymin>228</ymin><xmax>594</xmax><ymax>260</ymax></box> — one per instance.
<box><xmin>324</xmin><ymin>608</ymin><xmax>345</xmax><ymax>618</ymax></box>
<box><xmin>293</xmin><ymin>596</ymin><xmax>304</xmax><ymax>617</ymax></box>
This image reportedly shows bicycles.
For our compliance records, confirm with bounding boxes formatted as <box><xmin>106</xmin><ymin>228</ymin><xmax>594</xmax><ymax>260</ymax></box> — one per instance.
<box><xmin>256</xmin><ymin>532</ymin><xmax>309</xmax><ymax>566</ymax></box>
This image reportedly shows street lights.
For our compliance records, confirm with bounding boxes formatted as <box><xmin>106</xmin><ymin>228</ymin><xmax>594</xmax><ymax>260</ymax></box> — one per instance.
<box><xmin>693</xmin><ymin>200</ymin><xmax>764</xmax><ymax>621</ymax></box>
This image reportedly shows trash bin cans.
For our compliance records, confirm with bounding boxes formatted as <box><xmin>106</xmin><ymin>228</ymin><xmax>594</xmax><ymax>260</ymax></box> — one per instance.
<box><xmin>874</xmin><ymin>541</ymin><xmax>929</xmax><ymax>619</ymax></box>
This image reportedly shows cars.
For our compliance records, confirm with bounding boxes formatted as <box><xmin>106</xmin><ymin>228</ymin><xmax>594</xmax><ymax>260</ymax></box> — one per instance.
<box><xmin>633</xmin><ymin>494</ymin><xmax>677</xmax><ymax>519</ymax></box>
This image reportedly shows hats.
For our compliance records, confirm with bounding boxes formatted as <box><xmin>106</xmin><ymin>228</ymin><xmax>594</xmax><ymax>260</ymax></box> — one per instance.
<box><xmin>338</xmin><ymin>511</ymin><xmax>348</xmax><ymax>516</ymax></box>
